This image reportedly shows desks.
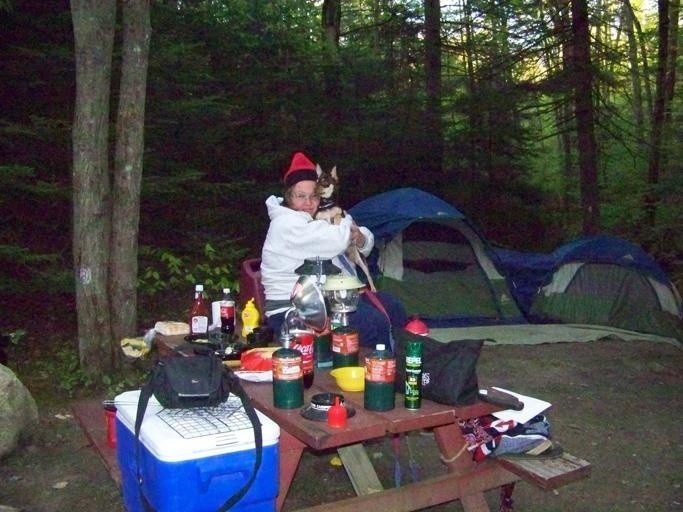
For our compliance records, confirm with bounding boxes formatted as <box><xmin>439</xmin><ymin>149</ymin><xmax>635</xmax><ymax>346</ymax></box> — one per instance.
<box><xmin>154</xmin><ymin>324</ymin><xmax>507</xmax><ymax>511</ymax></box>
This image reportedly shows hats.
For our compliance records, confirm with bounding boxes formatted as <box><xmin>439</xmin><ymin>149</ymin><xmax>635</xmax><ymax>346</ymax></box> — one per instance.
<box><xmin>284</xmin><ymin>152</ymin><xmax>318</xmax><ymax>187</ymax></box>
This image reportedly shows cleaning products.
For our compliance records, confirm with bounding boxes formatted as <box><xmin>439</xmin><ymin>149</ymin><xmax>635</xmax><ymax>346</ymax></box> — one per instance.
<box><xmin>272</xmin><ymin>332</ymin><xmax>307</xmax><ymax>407</ymax></box>
<box><xmin>362</xmin><ymin>344</ymin><xmax>397</xmax><ymax>409</ymax></box>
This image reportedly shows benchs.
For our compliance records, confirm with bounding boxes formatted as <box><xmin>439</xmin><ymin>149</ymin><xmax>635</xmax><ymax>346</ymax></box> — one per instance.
<box><xmin>71</xmin><ymin>395</ymin><xmax>131</xmax><ymax>508</ymax></box>
<box><xmin>487</xmin><ymin>449</ymin><xmax>593</xmax><ymax>512</ymax></box>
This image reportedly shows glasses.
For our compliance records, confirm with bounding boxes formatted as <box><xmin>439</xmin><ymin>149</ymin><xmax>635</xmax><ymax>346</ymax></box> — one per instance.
<box><xmin>292</xmin><ymin>191</ymin><xmax>318</xmax><ymax>199</ymax></box>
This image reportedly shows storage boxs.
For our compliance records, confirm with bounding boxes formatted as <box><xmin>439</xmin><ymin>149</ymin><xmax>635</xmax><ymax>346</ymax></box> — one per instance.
<box><xmin>112</xmin><ymin>388</ymin><xmax>281</xmax><ymax>512</ymax></box>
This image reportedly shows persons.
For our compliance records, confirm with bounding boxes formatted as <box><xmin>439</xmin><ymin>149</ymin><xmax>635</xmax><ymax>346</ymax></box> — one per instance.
<box><xmin>263</xmin><ymin>150</ymin><xmax>405</xmax><ymax>354</ymax></box>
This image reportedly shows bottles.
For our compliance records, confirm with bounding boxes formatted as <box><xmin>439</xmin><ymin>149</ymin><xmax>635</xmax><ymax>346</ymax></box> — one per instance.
<box><xmin>363</xmin><ymin>340</ymin><xmax>423</xmax><ymax>411</ymax></box>
<box><xmin>241</xmin><ymin>297</ymin><xmax>259</xmax><ymax>338</ymax></box>
<box><xmin>190</xmin><ymin>285</ymin><xmax>210</xmax><ymax>336</ymax></box>
<box><xmin>271</xmin><ymin>314</ymin><xmax>315</xmax><ymax>409</ymax></box>
<box><xmin>220</xmin><ymin>288</ymin><xmax>235</xmax><ymax>333</ymax></box>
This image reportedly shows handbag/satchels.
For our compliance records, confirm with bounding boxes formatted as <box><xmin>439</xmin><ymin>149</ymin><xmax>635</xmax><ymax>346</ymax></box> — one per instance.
<box><xmin>391</xmin><ymin>326</ymin><xmax>496</xmax><ymax>406</ymax></box>
<box><xmin>152</xmin><ymin>356</ymin><xmax>229</xmax><ymax>409</ymax></box>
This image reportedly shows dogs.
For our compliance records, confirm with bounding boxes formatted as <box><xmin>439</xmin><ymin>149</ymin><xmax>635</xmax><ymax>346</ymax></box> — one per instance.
<box><xmin>312</xmin><ymin>162</ymin><xmax>378</xmax><ymax>297</ymax></box>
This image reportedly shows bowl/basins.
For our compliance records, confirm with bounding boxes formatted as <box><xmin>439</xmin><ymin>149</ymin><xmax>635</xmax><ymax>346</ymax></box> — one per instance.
<box><xmin>330</xmin><ymin>366</ymin><xmax>364</xmax><ymax>392</ymax></box>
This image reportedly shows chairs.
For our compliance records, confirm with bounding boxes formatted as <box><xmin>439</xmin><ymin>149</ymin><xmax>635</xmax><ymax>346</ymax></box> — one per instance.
<box><xmin>241</xmin><ymin>257</ymin><xmax>391</xmax><ymax>326</ymax></box>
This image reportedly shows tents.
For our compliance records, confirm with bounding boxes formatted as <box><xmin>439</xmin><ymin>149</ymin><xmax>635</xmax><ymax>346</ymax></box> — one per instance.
<box><xmin>528</xmin><ymin>235</ymin><xmax>683</xmax><ymax>341</ymax></box>
<box><xmin>348</xmin><ymin>187</ymin><xmax>557</xmax><ymax>327</ymax></box>
